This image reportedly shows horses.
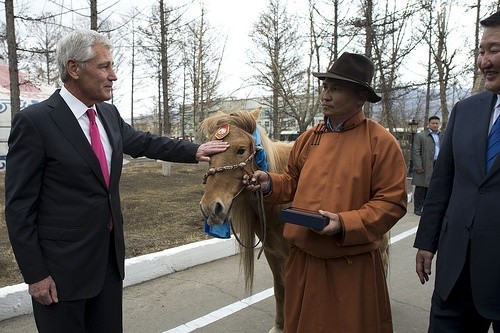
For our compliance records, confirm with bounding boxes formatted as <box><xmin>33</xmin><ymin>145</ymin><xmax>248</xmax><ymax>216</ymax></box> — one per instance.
<box><xmin>197</xmin><ymin>107</ymin><xmax>390</xmax><ymax>333</ymax></box>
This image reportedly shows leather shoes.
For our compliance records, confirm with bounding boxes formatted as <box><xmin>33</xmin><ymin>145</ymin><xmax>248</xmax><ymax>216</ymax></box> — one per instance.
<box><xmin>414</xmin><ymin>210</ymin><xmax>422</xmax><ymax>216</ymax></box>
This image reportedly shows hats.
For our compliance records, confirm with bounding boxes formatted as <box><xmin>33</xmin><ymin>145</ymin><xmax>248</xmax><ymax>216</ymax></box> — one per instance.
<box><xmin>311</xmin><ymin>52</ymin><xmax>383</xmax><ymax>104</ymax></box>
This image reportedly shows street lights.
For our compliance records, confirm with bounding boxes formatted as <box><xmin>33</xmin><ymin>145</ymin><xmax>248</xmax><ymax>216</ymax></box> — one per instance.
<box><xmin>407</xmin><ymin>116</ymin><xmax>419</xmax><ymax>178</ymax></box>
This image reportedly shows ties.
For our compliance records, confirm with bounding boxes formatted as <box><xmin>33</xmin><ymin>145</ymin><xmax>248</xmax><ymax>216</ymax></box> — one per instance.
<box><xmin>87</xmin><ymin>108</ymin><xmax>114</xmax><ymax>232</ymax></box>
<box><xmin>486</xmin><ymin>113</ymin><xmax>500</xmax><ymax>172</ymax></box>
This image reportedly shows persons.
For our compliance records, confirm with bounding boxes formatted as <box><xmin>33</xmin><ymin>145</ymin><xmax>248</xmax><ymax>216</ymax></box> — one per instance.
<box><xmin>413</xmin><ymin>116</ymin><xmax>445</xmax><ymax>216</ymax></box>
<box><xmin>411</xmin><ymin>11</ymin><xmax>500</xmax><ymax>333</ymax></box>
<box><xmin>5</xmin><ymin>30</ymin><xmax>230</xmax><ymax>333</ymax></box>
<box><xmin>241</xmin><ymin>52</ymin><xmax>409</xmax><ymax>333</ymax></box>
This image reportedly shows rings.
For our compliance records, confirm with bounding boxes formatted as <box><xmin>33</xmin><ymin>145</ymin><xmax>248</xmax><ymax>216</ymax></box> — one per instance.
<box><xmin>213</xmin><ymin>142</ymin><xmax>216</xmax><ymax>145</ymax></box>
